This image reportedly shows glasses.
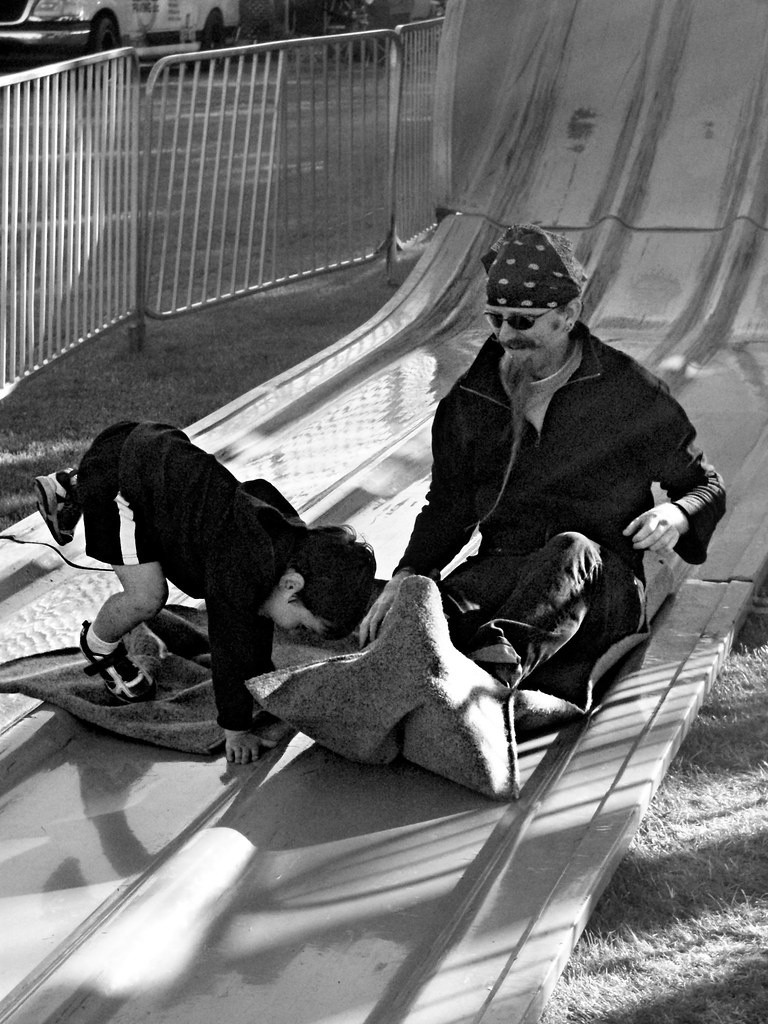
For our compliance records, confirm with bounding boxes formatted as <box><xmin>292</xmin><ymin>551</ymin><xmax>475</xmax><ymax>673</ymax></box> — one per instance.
<box><xmin>483</xmin><ymin>308</ymin><xmax>556</xmax><ymax>330</ymax></box>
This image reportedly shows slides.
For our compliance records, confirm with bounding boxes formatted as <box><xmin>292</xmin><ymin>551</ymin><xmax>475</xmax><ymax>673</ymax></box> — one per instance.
<box><xmin>0</xmin><ymin>2</ymin><xmax>768</xmax><ymax>1024</ymax></box>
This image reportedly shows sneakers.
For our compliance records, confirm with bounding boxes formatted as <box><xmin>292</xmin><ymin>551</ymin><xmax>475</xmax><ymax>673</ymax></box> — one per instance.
<box><xmin>80</xmin><ymin>621</ymin><xmax>157</xmax><ymax>704</ymax></box>
<box><xmin>35</xmin><ymin>467</ymin><xmax>82</xmax><ymax>547</ymax></box>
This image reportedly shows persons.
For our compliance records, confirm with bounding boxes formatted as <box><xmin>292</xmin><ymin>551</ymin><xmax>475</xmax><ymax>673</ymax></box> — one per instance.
<box><xmin>32</xmin><ymin>418</ymin><xmax>375</xmax><ymax>767</ymax></box>
<box><xmin>359</xmin><ymin>223</ymin><xmax>729</xmax><ymax>691</ymax></box>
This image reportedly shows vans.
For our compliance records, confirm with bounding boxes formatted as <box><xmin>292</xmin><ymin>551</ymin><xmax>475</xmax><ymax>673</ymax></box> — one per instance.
<box><xmin>1</xmin><ymin>0</ymin><xmax>242</xmax><ymax>88</ymax></box>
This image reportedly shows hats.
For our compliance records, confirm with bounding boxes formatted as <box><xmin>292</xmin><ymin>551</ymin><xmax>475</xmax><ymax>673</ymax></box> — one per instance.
<box><xmin>480</xmin><ymin>223</ymin><xmax>587</xmax><ymax>308</ymax></box>
